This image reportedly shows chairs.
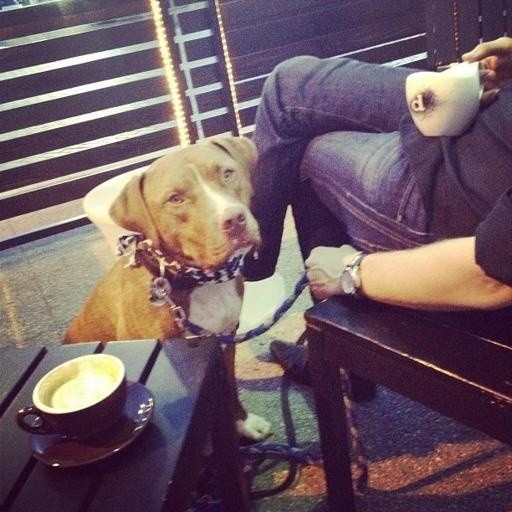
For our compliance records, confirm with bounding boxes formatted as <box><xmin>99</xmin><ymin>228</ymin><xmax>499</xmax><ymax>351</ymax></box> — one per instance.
<box><xmin>304</xmin><ymin>286</ymin><xmax>512</xmax><ymax>511</ymax></box>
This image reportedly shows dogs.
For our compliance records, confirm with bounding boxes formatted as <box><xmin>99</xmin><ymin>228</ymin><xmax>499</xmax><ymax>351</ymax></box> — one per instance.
<box><xmin>62</xmin><ymin>134</ymin><xmax>274</xmax><ymax>442</ymax></box>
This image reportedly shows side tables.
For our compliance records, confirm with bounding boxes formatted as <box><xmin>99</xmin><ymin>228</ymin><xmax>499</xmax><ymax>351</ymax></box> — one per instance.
<box><xmin>0</xmin><ymin>336</ymin><xmax>251</xmax><ymax>510</ymax></box>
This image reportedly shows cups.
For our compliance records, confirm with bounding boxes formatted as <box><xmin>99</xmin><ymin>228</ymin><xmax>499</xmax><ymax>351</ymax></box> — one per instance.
<box><xmin>405</xmin><ymin>62</ymin><xmax>486</xmax><ymax>139</ymax></box>
<box><xmin>14</xmin><ymin>353</ymin><xmax>126</xmax><ymax>437</ymax></box>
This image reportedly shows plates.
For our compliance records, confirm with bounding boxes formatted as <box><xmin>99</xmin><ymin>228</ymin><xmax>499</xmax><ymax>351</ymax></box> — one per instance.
<box><xmin>29</xmin><ymin>377</ymin><xmax>155</xmax><ymax>469</ymax></box>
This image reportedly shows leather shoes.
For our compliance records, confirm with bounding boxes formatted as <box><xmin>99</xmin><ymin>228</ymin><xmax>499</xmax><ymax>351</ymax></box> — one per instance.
<box><xmin>270</xmin><ymin>339</ymin><xmax>376</xmax><ymax>400</ymax></box>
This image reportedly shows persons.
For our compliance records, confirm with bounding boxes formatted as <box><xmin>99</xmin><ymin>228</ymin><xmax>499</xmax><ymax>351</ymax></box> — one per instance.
<box><xmin>236</xmin><ymin>36</ymin><xmax>511</xmax><ymax>395</ymax></box>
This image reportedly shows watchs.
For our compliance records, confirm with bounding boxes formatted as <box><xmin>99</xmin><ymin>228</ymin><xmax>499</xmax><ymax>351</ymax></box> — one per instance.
<box><xmin>339</xmin><ymin>253</ymin><xmax>367</xmax><ymax>301</ymax></box>
